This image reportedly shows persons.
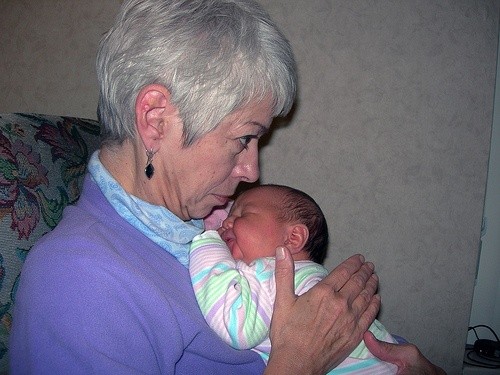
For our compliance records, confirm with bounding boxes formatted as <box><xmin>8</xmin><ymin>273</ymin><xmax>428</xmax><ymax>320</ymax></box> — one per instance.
<box><xmin>186</xmin><ymin>183</ymin><xmax>403</xmax><ymax>375</ymax></box>
<box><xmin>4</xmin><ymin>0</ymin><xmax>448</xmax><ymax>374</ymax></box>
